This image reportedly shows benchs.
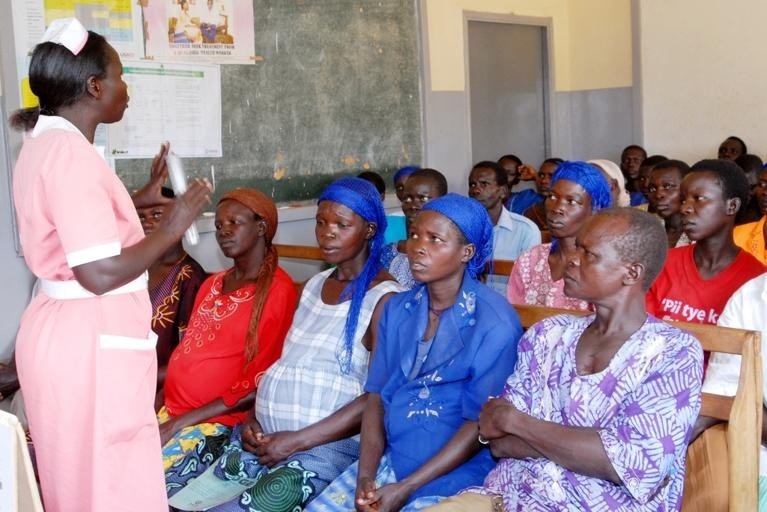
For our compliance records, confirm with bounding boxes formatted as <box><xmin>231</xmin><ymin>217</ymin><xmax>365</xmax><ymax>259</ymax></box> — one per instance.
<box><xmin>274</xmin><ymin>243</ymin><xmax>515</xmax><ymax>276</ymax></box>
<box><xmin>515</xmin><ymin>306</ymin><xmax>763</xmax><ymax>511</ymax></box>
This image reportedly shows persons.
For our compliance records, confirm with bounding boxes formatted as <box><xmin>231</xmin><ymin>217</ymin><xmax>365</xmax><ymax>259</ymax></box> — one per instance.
<box><xmin>418</xmin><ymin>206</ymin><xmax>706</xmax><ymax>512</ymax></box>
<box><xmin>173</xmin><ymin>0</ymin><xmax>196</xmax><ymax>44</ymax></box>
<box><xmin>165</xmin><ymin>173</ymin><xmax>411</xmax><ymax>512</ymax></box>
<box><xmin>356</xmin><ymin>134</ymin><xmax>767</xmax><ymax>437</ymax></box>
<box><xmin>199</xmin><ymin>1</ymin><xmax>219</xmax><ymax>28</ymax></box>
<box><xmin>154</xmin><ymin>185</ymin><xmax>299</xmax><ymax>467</ymax></box>
<box><xmin>300</xmin><ymin>191</ymin><xmax>522</xmax><ymax>512</ymax></box>
<box><xmin>131</xmin><ymin>183</ymin><xmax>206</xmax><ymax>386</ymax></box>
<box><xmin>13</xmin><ymin>16</ymin><xmax>213</xmax><ymax>510</ymax></box>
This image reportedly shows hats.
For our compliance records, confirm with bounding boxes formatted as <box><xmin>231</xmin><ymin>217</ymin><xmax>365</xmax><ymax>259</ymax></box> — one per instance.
<box><xmin>35</xmin><ymin>17</ymin><xmax>89</xmax><ymax>56</ymax></box>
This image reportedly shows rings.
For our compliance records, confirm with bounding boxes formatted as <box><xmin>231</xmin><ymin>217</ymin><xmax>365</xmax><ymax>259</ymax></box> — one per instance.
<box><xmin>478</xmin><ymin>436</ymin><xmax>490</xmax><ymax>445</ymax></box>
